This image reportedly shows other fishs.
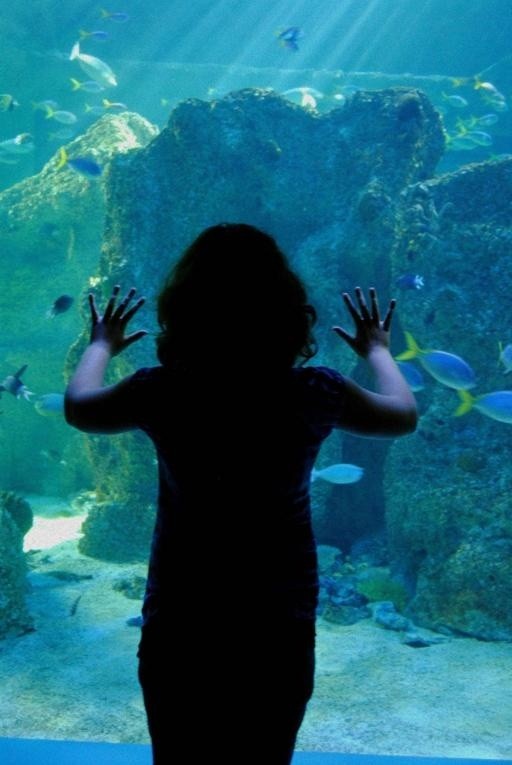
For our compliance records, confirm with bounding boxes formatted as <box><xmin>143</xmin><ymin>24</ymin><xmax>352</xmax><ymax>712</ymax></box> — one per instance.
<box><xmin>78</xmin><ymin>29</ymin><xmax>108</xmax><ymax>41</ymax></box>
<box><xmin>0</xmin><ymin>364</ymin><xmax>36</xmax><ymax>402</ymax></box>
<box><xmin>395</xmin><ymin>273</ymin><xmax>424</xmax><ymax>291</ymax></box>
<box><xmin>207</xmin><ymin>86</ymin><xmax>225</xmax><ymax>99</ymax></box>
<box><xmin>0</xmin><ymin>78</ymin><xmax>130</xmax><ymax>180</ymax></box>
<box><xmin>496</xmin><ymin>341</ymin><xmax>512</xmax><ymax>376</ymax></box>
<box><xmin>284</xmin><ymin>87</ymin><xmax>325</xmax><ymax>99</ymax></box>
<box><xmin>101</xmin><ymin>9</ymin><xmax>128</xmax><ymax>23</ymax></box>
<box><xmin>277</xmin><ymin>26</ymin><xmax>300</xmax><ymax>42</ymax></box>
<box><xmin>33</xmin><ymin>392</ymin><xmax>70</xmax><ymax>419</ymax></box>
<box><xmin>33</xmin><ymin>222</ymin><xmax>60</xmax><ymax>236</ymax></box>
<box><xmin>394</xmin><ymin>329</ymin><xmax>479</xmax><ymax>392</ymax></box>
<box><xmin>47</xmin><ymin>294</ymin><xmax>74</xmax><ymax>317</ymax></box>
<box><xmin>160</xmin><ymin>96</ymin><xmax>183</xmax><ymax>109</ymax></box>
<box><xmin>454</xmin><ymin>388</ymin><xmax>512</xmax><ymax>425</ymax></box>
<box><xmin>68</xmin><ymin>41</ymin><xmax>117</xmax><ymax>88</ymax></box>
<box><xmin>309</xmin><ymin>462</ymin><xmax>364</xmax><ymax>485</ymax></box>
<box><xmin>392</xmin><ymin>361</ymin><xmax>425</xmax><ymax>391</ymax></box>
<box><xmin>432</xmin><ymin>75</ymin><xmax>508</xmax><ymax>151</ymax></box>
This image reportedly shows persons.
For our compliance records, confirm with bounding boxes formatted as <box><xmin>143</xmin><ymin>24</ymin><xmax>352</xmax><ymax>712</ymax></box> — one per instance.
<box><xmin>61</xmin><ymin>224</ymin><xmax>420</xmax><ymax>765</ymax></box>
